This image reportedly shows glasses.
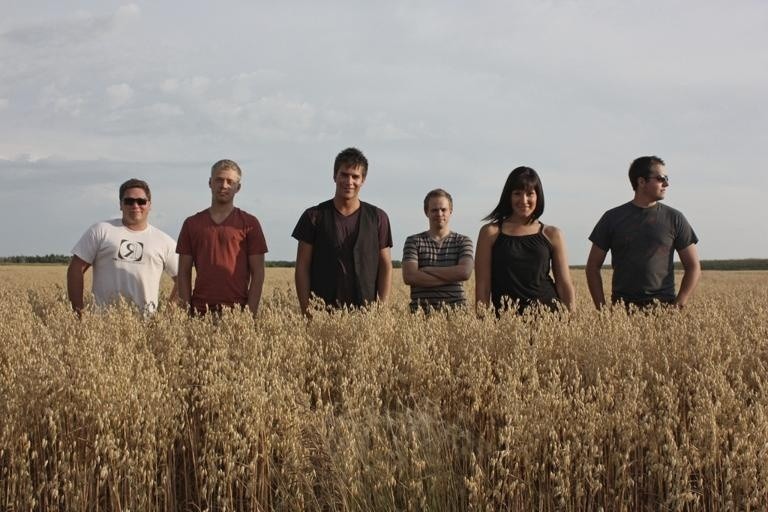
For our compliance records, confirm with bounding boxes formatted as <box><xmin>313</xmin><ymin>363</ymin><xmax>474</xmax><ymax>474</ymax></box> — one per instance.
<box><xmin>645</xmin><ymin>174</ymin><xmax>669</xmax><ymax>182</ymax></box>
<box><xmin>123</xmin><ymin>198</ymin><xmax>147</xmax><ymax>206</ymax></box>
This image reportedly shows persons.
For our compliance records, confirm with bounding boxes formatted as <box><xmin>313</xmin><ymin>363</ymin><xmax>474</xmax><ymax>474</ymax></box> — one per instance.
<box><xmin>67</xmin><ymin>178</ymin><xmax>180</xmax><ymax>319</ymax></box>
<box><xmin>475</xmin><ymin>166</ymin><xmax>576</xmax><ymax>320</ymax></box>
<box><xmin>586</xmin><ymin>155</ymin><xmax>701</xmax><ymax>317</ymax></box>
<box><xmin>175</xmin><ymin>159</ymin><xmax>268</xmax><ymax>317</ymax></box>
<box><xmin>400</xmin><ymin>188</ymin><xmax>474</xmax><ymax>316</ymax></box>
<box><xmin>291</xmin><ymin>147</ymin><xmax>394</xmax><ymax>321</ymax></box>
